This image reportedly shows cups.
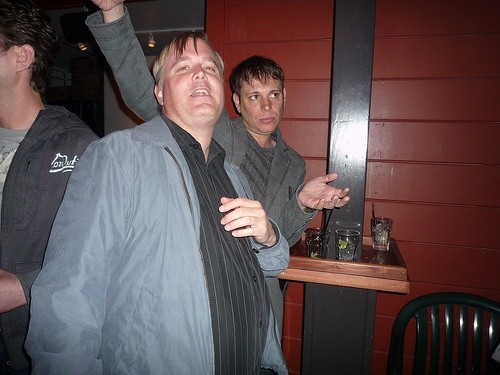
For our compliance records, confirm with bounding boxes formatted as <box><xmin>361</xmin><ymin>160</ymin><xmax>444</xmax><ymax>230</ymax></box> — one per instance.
<box><xmin>307</xmin><ymin>227</ymin><xmax>330</xmax><ymax>259</ymax></box>
<box><xmin>371</xmin><ymin>216</ymin><xmax>393</xmax><ymax>251</ymax></box>
<box><xmin>335</xmin><ymin>229</ymin><xmax>361</xmax><ymax>261</ymax></box>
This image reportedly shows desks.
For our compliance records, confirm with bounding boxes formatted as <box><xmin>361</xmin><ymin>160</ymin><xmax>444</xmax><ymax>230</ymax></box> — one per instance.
<box><xmin>276</xmin><ymin>239</ymin><xmax>412</xmax><ymax>294</ymax></box>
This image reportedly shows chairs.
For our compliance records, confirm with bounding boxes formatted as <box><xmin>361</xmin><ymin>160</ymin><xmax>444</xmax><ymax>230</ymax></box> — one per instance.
<box><xmin>386</xmin><ymin>292</ymin><xmax>500</xmax><ymax>375</ymax></box>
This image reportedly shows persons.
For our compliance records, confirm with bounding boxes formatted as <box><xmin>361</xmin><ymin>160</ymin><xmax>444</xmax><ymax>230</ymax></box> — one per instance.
<box><xmin>24</xmin><ymin>31</ymin><xmax>290</xmax><ymax>375</ymax></box>
<box><xmin>85</xmin><ymin>0</ymin><xmax>350</xmax><ymax>346</ymax></box>
<box><xmin>0</xmin><ymin>0</ymin><xmax>99</xmax><ymax>375</ymax></box>
